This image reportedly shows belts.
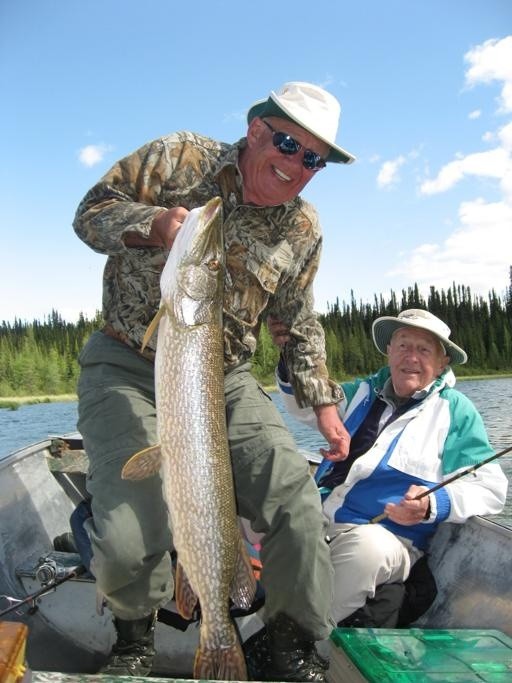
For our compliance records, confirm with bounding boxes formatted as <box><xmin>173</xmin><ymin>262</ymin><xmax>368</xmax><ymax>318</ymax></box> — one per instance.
<box><xmin>99</xmin><ymin>322</ymin><xmax>121</xmax><ymax>340</ymax></box>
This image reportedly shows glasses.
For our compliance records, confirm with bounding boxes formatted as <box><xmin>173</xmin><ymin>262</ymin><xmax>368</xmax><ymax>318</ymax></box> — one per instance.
<box><xmin>264</xmin><ymin>121</ymin><xmax>326</xmax><ymax>172</ymax></box>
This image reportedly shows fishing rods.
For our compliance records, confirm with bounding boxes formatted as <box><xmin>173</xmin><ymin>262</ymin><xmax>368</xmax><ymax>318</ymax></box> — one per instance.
<box><xmin>369</xmin><ymin>442</ymin><xmax>512</xmax><ymax>522</ymax></box>
<box><xmin>0</xmin><ymin>554</ymin><xmax>86</xmax><ymax>620</ymax></box>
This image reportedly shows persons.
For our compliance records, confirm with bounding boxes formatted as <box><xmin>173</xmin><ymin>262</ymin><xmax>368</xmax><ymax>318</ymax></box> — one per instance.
<box><xmin>278</xmin><ymin>308</ymin><xmax>510</xmax><ymax>626</ymax></box>
<box><xmin>70</xmin><ymin>79</ymin><xmax>351</xmax><ymax>682</ymax></box>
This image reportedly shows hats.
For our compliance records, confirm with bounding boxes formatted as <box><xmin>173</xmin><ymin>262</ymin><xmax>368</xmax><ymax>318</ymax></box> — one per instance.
<box><xmin>371</xmin><ymin>309</ymin><xmax>468</xmax><ymax>366</ymax></box>
<box><xmin>247</xmin><ymin>81</ymin><xmax>356</xmax><ymax>164</ymax></box>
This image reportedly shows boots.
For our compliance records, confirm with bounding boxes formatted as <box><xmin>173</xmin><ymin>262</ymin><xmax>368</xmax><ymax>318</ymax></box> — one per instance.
<box><xmin>94</xmin><ymin>613</ymin><xmax>157</xmax><ymax>676</ymax></box>
<box><xmin>242</xmin><ymin>613</ymin><xmax>330</xmax><ymax>682</ymax></box>
